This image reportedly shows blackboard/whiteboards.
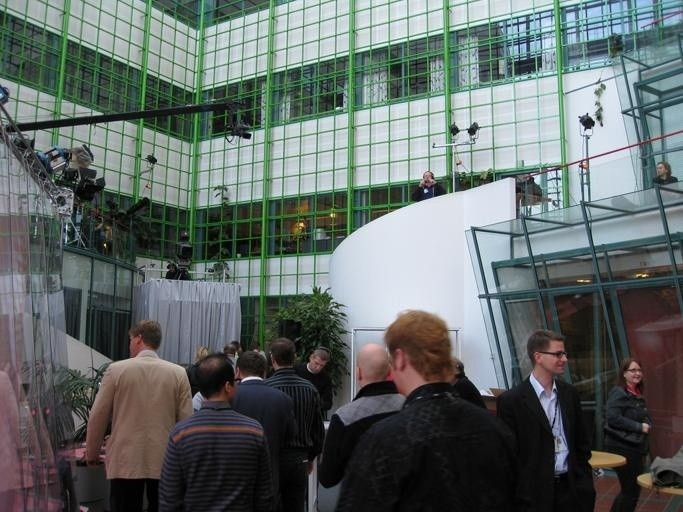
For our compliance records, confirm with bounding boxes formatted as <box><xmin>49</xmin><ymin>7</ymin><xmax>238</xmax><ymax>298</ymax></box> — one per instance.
<box><xmin>351</xmin><ymin>327</ymin><xmax>461</xmax><ymax>401</ymax></box>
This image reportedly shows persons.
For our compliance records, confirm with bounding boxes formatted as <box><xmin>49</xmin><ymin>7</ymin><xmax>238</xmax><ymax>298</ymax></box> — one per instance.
<box><xmin>84</xmin><ymin>311</ymin><xmax>195</xmax><ymax>512</ymax></box>
<box><xmin>336</xmin><ymin>310</ymin><xmax>533</xmax><ymax>511</ymax></box>
<box><xmin>600</xmin><ymin>355</ymin><xmax>654</xmax><ymax>512</ymax></box>
<box><xmin>441</xmin><ymin>356</ymin><xmax>493</xmax><ymax>415</ymax></box>
<box><xmin>495</xmin><ymin>330</ymin><xmax>597</xmax><ymax>512</ymax></box>
<box><xmin>0</xmin><ymin>359</ymin><xmax>80</xmax><ymax>512</ymax></box>
<box><xmin>411</xmin><ymin>170</ymin><xmax>448</xmax><ymax>203</ymax></box>
<box><xmin>164</xmin><ymin>263</ymin><xmax>187</xmax><ymax>281</ymax></box>
<box><xmin>312</xmin><ymin>340</ymin><xmax>408</xmax><ymax>512</ymax></box>
<box><xmin>156</xmin><ymin>338</ymin><xmax>336</xmax><ymax>512</ymax></box>
<box><xmin>649</xmin><ymin>161</ymin><xmax>681</xmax><ymax>203</ymax></box>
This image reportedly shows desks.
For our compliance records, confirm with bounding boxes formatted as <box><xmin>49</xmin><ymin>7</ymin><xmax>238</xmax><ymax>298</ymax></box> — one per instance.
<box><xmin>586</xmin><ymin>448</ymin><xmax>682</xmax><ymax>497</ymax></box>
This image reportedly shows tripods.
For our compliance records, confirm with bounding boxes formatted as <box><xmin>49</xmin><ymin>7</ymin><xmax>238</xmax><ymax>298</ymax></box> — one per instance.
<box><xmin>174</xmin><ymin>268</ymin><xmax>194</xmax><ymax>281</ymax></box>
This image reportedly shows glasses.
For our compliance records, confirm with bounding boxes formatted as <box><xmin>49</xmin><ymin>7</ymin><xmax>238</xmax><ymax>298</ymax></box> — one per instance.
<box><xmin>542</xmin><ymin>351</ymin><xmax>568</xmax><ymax>358</ymax></box>
<box><xmin>627</xmin><ymin>368</ymin><xmax>644</xmax><ymax>374</ymax></box>
